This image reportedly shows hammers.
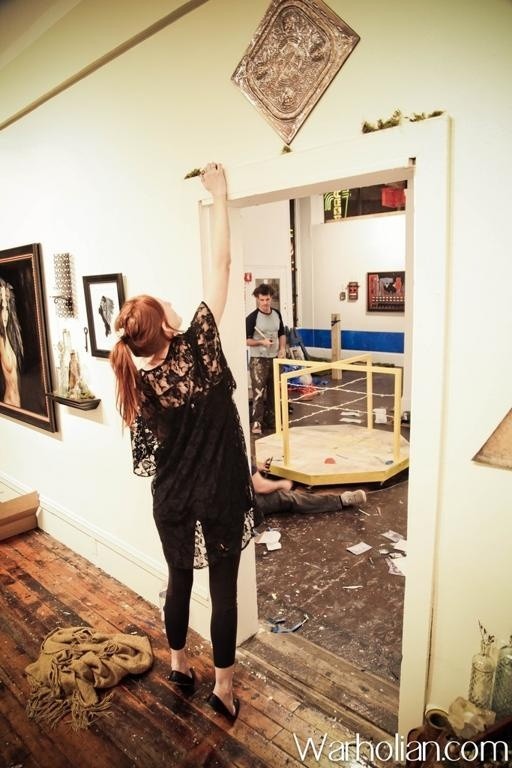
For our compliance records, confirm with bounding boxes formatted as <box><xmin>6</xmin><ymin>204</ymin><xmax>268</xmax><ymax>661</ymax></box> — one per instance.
<box><xmin>254</xmin><ymin>327</ymin><xmax>273</xmax><ymax>344</ymax></box>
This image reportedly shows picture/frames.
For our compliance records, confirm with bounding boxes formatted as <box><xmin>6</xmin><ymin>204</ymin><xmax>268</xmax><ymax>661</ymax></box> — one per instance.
<box><xmin>1</xmin><ymin>242</ymin><xmax>59</xmax><ymax>434</ymax></box>
<box><xmin>83</xmin><ymin>272</ymin><xmax>127</xmax><ymax>358</ymax></box>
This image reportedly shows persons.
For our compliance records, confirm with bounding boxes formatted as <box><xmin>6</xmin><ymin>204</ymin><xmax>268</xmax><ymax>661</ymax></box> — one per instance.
<box><xmin>246</xmin><ymin>283</ymin><xmax>288</xmax><ymax>434</ymax></box>
<box><xmin>109</xmin><ymin>162</ymin><xmax>253</xmax><ymax>724</ymax></box>
<box><xmin>249</xmin><ymin>442</ymin><xmax>366</xmax><ymax>524</ymax></box>
<box><xmin>1</xmin><ymin>278</ymin><xmax>28</xmax><ymax>408</ymax></box>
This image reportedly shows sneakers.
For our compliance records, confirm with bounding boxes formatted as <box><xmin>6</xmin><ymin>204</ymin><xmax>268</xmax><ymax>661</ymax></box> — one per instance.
<box><xmin>208</xmin><ymin>692</ymin><xmax>241</xmax><ymax>722</ymax></box>
<box><xmin>169</xmin><ymin>666</ymin><xmax>195</xmax><ymax>688</ymax></box>
<box><xmin>251</xmin><ymin>420</ymin><xmax>262</xmax><ymax>434</ymax></box>
<box><xmin>341</xmin><ymin>490</ymin><xmax>366</xmax><ymax>508</ymax></box>
